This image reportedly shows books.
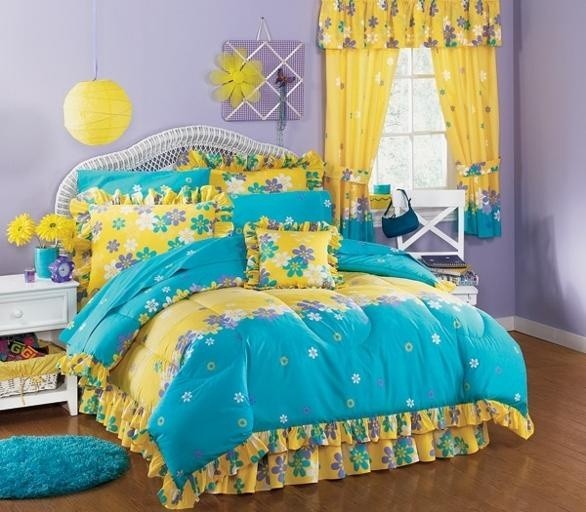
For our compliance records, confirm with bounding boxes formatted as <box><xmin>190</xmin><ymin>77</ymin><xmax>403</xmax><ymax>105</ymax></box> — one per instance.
<box><xmin>417</xmin><ymin>252</ymin><xmax>467</xmax><ymax>285</ymax></box>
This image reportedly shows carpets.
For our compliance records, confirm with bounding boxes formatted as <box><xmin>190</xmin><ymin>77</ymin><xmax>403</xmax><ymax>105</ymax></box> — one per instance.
<box><xmin>0</xmin><ymin>435</ymin><xmax>135</xmax><ymax>502</ymax></box>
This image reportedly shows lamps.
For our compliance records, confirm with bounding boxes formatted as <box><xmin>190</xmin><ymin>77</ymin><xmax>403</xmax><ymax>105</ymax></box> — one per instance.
<box><xmin>60</xmin><ymin>1</ymin><xmax>135</xmax><ymax>147</ymax></box>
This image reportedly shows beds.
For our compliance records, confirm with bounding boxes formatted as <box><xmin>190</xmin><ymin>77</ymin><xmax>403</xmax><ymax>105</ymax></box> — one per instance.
<box><xmin>52</xmin><ymin>126</ymin><xmax>536</xmax><ymax>512</ymax></box>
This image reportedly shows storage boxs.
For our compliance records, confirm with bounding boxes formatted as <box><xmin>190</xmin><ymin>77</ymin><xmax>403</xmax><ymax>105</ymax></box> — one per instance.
<box><xmin>367</xmin><ymin>193</ymin><xmax>391</xmax><ymax>209</ymax></box>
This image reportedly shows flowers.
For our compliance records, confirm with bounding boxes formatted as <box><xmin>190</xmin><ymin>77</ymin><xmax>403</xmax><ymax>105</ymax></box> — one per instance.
<box><xmin>209</xmin><ymin>48</ymin><xmax>263</xmax><ymax>111</ymax></box>
<box><xmin>6</xmin><ymin>213</ymin><xmax>81</xmax><ymax>256</ymax></box>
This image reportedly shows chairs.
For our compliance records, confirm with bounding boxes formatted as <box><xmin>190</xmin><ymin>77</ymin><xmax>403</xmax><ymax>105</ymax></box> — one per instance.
<box><xmin>394</xmin><ymin>187</ymin><xmax>480</xmax><ymax>308</ymax></box>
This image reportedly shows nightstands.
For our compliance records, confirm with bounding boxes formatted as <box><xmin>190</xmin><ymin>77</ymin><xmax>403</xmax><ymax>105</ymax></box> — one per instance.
<box><xmin>0</xmin><ymin>271</ymin><xmax>82</xmax><ymax>422</ymax></box>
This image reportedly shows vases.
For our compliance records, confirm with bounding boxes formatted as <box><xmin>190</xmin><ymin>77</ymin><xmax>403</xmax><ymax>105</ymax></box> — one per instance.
<box><xmin>31</xmin><ymin>246</ymin><xmax>61</xmax><ymax>281</ymax></box>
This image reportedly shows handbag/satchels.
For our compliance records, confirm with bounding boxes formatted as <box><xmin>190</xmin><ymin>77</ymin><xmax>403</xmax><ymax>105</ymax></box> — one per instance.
<box><xmin>382</xmin><ymin>209</ymin><xmax>418</xmax><ymax>239</ymax></box>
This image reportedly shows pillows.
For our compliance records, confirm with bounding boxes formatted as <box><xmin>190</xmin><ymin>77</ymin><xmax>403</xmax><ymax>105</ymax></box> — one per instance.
<box><xmin>69</xmin><ymin>186</ymin><xmax>234</xmax><ymax>308</ymax></box>
<box><xmin>226</xmin><ymin>189</ymin><xmax>338</xmax><ymax>233</ymax></box>
<box><xmin>240</xmin><ymin>217</ymin><xmax>344</xmax><ymax>291</ymax></box>
<box><xmin>168</xmin><ymin>146</ymin><xmax>327</xmax><ymax>197</ymax></box>
<box><xmin>71</xmin><ymin>163</ymin><xmax>212</xmax><ymax>194</ymax></box>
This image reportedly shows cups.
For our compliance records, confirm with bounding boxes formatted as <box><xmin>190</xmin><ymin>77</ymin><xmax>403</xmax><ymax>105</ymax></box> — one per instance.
<box><xmin>24</xmin><ymin>270</ymin><xmax>35</xmax><ymax>284</ymax></box>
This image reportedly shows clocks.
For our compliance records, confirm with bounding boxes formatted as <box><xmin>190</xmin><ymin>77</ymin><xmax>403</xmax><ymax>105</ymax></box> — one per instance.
<box><xmin>48</xmin><ymin>254</ymin><xmax>76</xmax><ymax>283</ymax></box>
